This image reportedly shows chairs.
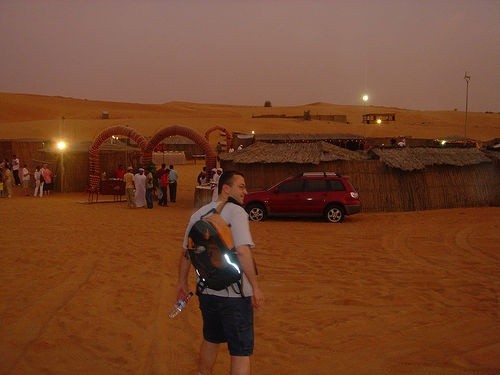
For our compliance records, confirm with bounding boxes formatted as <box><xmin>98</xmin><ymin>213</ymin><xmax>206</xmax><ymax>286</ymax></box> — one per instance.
<box><xmin>88</xmin><ymin>179</ymin><xmax>126</xmax><ymax>202</ymax></box>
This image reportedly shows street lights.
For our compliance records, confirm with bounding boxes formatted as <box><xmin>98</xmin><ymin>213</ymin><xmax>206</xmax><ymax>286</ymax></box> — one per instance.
<box><xmin>57</xmin><ymin>142</ymin><xmax>66</xmax><ymax>192</ymax></box>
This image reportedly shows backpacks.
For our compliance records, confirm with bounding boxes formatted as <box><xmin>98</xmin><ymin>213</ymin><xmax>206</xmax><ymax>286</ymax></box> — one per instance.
<box><xmin>39</xmin><ymin>174</ymin><xmax>44</xmax><ymax>183</ymax></box>
<box><xmin>187</xmin><ymin>196</ymin><xmax>241</xmax><ymax>296</ymax></box>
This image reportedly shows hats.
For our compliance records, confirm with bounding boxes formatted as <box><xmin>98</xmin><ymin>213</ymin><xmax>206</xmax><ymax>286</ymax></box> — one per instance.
<box><xmin>127</xmin><ymin>167</ymin><xmax>133</xmax><ymax>170</ymax></box>
<box><xmin>212</xmin><ymin>168</ymin><xmax>217</xmax><ymax>173</ymax></box>
<box><xmin>217</xmin><ymin>168</ymin><xmax>222</xmax><ymax>172</ymax></box>
<box><xmin>138</xmin><ymin>168</ymin><xmax>144</xmax><ymax>170</ymax></box>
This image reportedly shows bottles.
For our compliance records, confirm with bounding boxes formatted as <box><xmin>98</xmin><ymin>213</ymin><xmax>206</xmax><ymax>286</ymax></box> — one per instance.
<box><xmin>168</xmin><ymin>291</ymin><xmax>194</xmax><ymax>318</ymax></box>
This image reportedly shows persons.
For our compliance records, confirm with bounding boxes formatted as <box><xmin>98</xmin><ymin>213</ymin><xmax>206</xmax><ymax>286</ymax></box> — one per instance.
<box><xmin>0</xmin><ymin>137</ymin><xmax>475</xmax><ymax>210</ymax></box>
<box><xmin>176</xmin><ymin>170</ymin><xmax>265</xmax><ymax>375</ymax></box>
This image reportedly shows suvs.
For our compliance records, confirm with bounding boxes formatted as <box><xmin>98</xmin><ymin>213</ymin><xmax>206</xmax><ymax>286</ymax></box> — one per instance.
<box><xmin>244</xmin><ymin>170</ymin><xmax>363</xmax><ymax>223</ymax></box>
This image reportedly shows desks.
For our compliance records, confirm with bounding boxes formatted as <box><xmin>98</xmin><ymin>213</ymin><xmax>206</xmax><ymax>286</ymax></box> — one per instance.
<box><xmin>192</xmin><ymin>184</ymin><xmax>214</xmax><ymax>209</ymax></box>
